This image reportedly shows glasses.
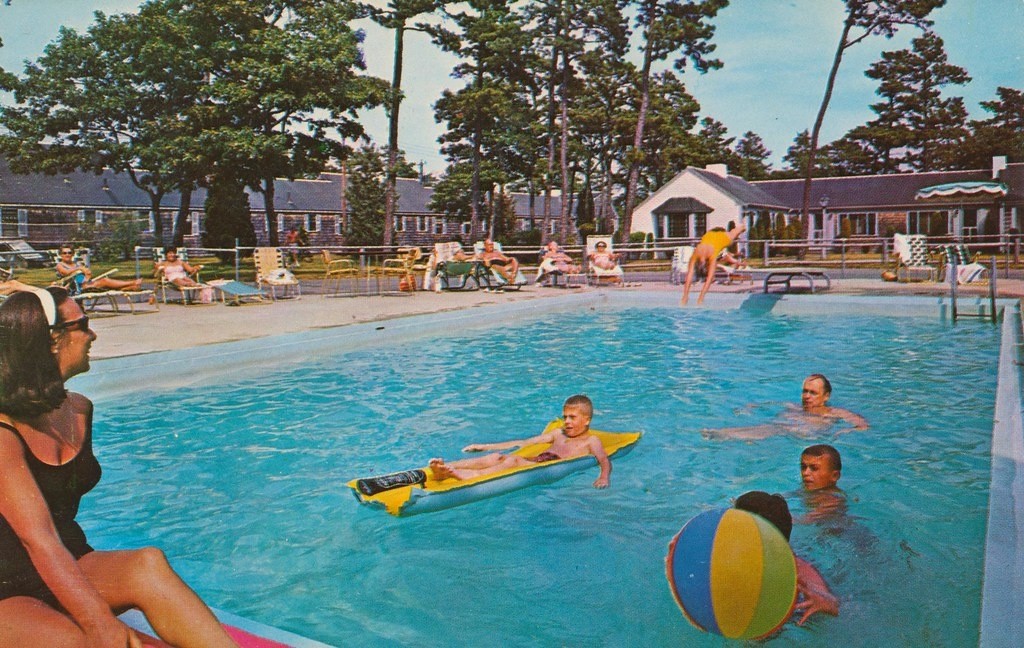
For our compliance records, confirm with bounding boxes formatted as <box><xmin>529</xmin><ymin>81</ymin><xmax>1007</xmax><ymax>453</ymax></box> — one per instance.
<box><xmin>599</xmin><ymin>246</ymin><xmax>605</xmax><ymax>248</ymax></box>
<box><xmin>62</xmin><ymin>251</ymin><xmax>73</xmax><ymax>254</ymax></box>
<box><xmin>46</xmin><ymin>316</ymin><xmax>90</xmax><ymax>332</ymax></box>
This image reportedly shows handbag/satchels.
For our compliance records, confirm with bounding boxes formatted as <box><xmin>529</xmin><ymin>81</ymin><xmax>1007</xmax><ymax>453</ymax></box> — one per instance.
<box><xmin>398</xmin><ymin>273</ymin><xmax>418</xmax><ymax>291</ymax></box>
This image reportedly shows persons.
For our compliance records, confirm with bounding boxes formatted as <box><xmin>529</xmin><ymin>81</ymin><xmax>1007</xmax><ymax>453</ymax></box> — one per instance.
<box><xmin>56</xmin><ymin>225</ymin><xmax>750</xmax><ymax>296</ymax></box>
<box><xmin>428</xmin><ymin>394</ymin><xmax>611</xmax><ymax>490</ymax></box>
<box><xmin>698</xmin><ymin>373</ymin><xmax>870</xmax><ymax>445</ymax></box>
<box><xmin>0</xmin><ymin>284</ymin><xmax>238</xmax><ymax>648</ymax></box>
<box><xmin>782</xmin><ymin>445</ymin><xmax>849</xmax><ymax>524</ymax></box>
<box><xmin>727</xmin><ymin>490</ymin><xmax>838</xmax><ymax>627</ymax></box>
<box><xmin>681</xmin><ymin>225</ymin><xmax>746</xmax><ymax>307</ymax></box>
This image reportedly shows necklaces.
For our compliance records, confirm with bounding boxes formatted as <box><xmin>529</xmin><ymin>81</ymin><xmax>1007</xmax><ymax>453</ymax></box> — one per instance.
<box><xmin>45</xmin><ymin>390</ymin><xmax>75</xmax><ymax>445</ymax></box>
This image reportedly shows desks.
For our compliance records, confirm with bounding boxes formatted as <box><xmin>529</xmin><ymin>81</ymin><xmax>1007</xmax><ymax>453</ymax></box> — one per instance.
<box><xmin>335</xmin><ymin>251</ymin><xmax>408</xmax><ymax>296</ymax></box>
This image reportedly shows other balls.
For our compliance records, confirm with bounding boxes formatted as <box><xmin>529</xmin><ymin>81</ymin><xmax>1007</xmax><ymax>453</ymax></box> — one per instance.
<box><xmin>664</xmin><ymin>506</ymin><xmax>800</xmax><ymax>641</ymax></box>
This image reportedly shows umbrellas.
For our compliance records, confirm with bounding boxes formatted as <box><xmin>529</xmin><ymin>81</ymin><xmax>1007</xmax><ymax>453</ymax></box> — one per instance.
<box><xmin>915</xmin><ymin>178</ymin><xmax>1009</xmax><ymax>246</ymax></box>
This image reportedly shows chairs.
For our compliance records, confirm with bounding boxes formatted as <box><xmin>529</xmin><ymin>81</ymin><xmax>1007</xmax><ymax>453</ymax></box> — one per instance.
<box><xmin>253</xmin><ymin>247</ymin><xmax>302</xmax><ymax>300</ymax></box>
<box><xmin>49</xmin><ymin>248</ymin><xmax>158</xmax><ymax>318</ymax></box>
<box><xmin>193</xmin><ymin>257</ymin><xmax>271</xmax><ymax>305</ymax></box>
<box><xmin>422</xmin><ymin>241</ymin><xmax>528</xmax><ymax>291</ymax></box>
<box><xmin>582</xmin><ymin>235</ymin><xmax>626</xmax><ymax>286</ymax></box>
<box><xmin>149</xmin><ymin>247</ymin><xmax>210</xmax><ymax>305</ymax></box>
<box><xmin>320</xmin><ymin>249</ymin><xmax>419</xmax><ymax>298</ymax></box>
<box><xmin>536</xmin><ymin>246</ymin><xmax>580</xmax><ymax>289</ymax></box>
<box><xmin>672</xmin><ymin>246</ymin><xmax>753</xmax><ymax>287</ymax></box>
<box><xmin>891</xmin><ymin>233</ymin><xmax>989</xmax><ymax>287</ymax></box>
